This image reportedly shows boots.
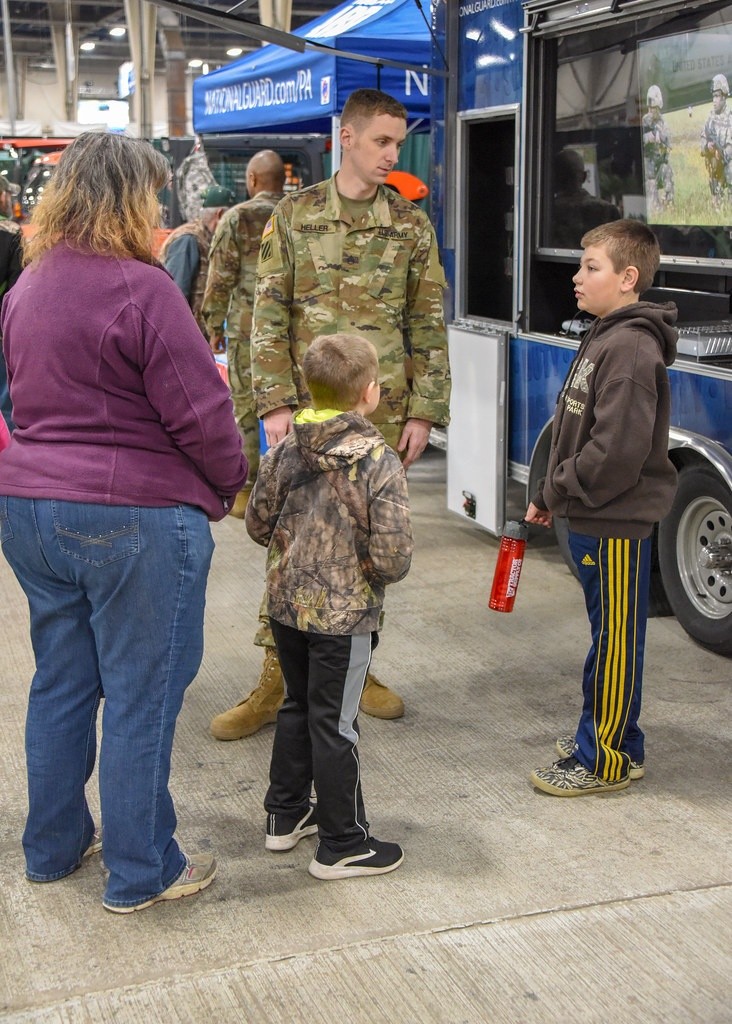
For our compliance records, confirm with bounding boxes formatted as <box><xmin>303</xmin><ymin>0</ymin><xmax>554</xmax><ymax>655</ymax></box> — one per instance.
<box><xmin>358</xmin><ymin>673</ymin><xmax>405</xmax><ymax>722</ymax></box>
<box><xmin>227</xmin><ymin>490</ymin><xmax>254</xmax><ymax>519</ymax></box>
<box><xmin>210</xmin><ymin>645</ymin><xmax>286</xmax><ymax>742</ymax></box>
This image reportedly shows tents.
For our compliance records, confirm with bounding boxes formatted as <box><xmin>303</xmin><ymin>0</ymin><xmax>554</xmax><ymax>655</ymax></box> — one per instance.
<box><xmin>193</xmin><ymin>0</ymin><xmax>433</xmax><ymax>217</ymax></box>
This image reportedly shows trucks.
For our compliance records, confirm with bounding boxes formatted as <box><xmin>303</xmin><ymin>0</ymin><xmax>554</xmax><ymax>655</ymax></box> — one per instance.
<box><xmin>429</xmin><ymin>0</ymin><xmax>732</xmax><ymax>662</ymax></box>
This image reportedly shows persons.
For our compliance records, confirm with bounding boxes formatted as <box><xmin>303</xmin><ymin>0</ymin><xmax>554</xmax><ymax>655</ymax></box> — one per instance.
<box><xmin>0</xmin><ymin>220</ymin><xmax>26</xmax><ymax>437</ymax></box>
<box><xmin>201</xmin><ymin>150</ymin><xmax>287</xmax><ymax>519</ymax></box>
<box><xmin>208</xmin><ymin>88</ymin><xmax>453</xmax><ymax>742</ymax></box>
<box><xmin>522</xmin><ymin>219</ymin><xmax>680</xmax><ymax>799</ymax></box>
<box><xmin>0</xmin><ymin>130</ymin><xmax>249</xmax><ymax>915</ymax></box>
<box><xmin>242</xmin><ymin>336</ymin><xmax>414</xmax><ymax>879</ymax></box>
<box><xmin>641</xmin><ymin>85</ymin><xmax>675</xmax><ymax>218</ymax></box>
<box><xmin>550</xmin><ymin>148</ymin><xmax>622</xmax><ymax>336</ymax></box>
<box><xmin>700</xmin><ymin>74</ymin><xmax>732</xmax><ymax>212</ymax></box>
<box><xmin>157</xmin><ymin>186</ymin><xmax>237</xmax><ymax>344</ymax></box>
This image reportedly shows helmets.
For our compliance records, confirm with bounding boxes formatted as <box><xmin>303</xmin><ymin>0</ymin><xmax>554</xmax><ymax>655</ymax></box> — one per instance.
<box><xmin>710</xmin><ymin>73</ymin><xmax>730</xmax><ymax>96</ymax></box>
<box><xmin>646</xmin><ymin>84</ymin><xmax>663</xmax><ymax>110</ymax></box>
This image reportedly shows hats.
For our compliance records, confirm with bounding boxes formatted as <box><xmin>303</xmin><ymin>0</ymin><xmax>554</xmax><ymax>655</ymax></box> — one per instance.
<box><xmin>202</xmin><ymin>186</ymin><xmax>238</xmax><ymax>207</ymax></box>
<box><xmin>0</xmin><ymin>174</ymin><xmax>21</xmax><ymax>194</ymax></box>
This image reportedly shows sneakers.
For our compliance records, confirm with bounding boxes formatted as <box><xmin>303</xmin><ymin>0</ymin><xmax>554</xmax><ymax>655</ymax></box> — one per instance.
<box><xmin>265</xmin><ymin>795</ymin><xmax>318</xmax><ymax>852</ymax></box>
<box><xmin>86</xmin><ymin>825</ymin><xmax>104</xmax><ymax>857</ymax></box>
<box><xmin>531</xmin><ymin>756</ymin><xmax>628</xmax><ymax>798</ymax></box>
<box><xmin>558</xmin><ymin>732</ymin><xmax>646</xmax><ymax>779</ymax></box>
<box><xmin>103</xmin><ymin>851</ymin><xmax>216</xmax><ymax>914</ymax></box>
<box><xmin>309</xmin><ymin>837</ymin><xmax>404</xmax><ymax>881</ymax></box>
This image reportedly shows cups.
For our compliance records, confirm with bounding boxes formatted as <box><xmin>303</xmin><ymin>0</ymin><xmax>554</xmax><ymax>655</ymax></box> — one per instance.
<box><xmin>489</xmin><ymin>520</ymin><xmax>529</xmax><ymax>614</ymax></box>
